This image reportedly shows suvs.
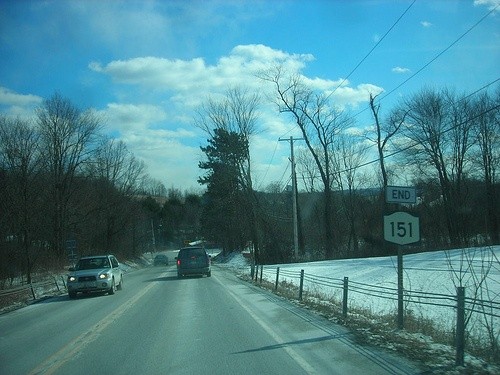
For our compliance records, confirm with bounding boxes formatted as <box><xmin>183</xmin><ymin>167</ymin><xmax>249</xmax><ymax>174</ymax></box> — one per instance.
<box><xmin>176</xmin><ymin>247</ymin><xmax>212</xmax><ymax>278</ymax></box>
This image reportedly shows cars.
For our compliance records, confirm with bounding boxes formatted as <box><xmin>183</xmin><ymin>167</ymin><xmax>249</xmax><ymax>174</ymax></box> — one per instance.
<box><xmin>154</xmin><ymin>255</ymin><xmax>168</xmax><ymax>266</ymax></box>
<box><xmin>67</xmin><ymin>255</ymin><xmax>123</xmax><ymax>298</ymax></box>
